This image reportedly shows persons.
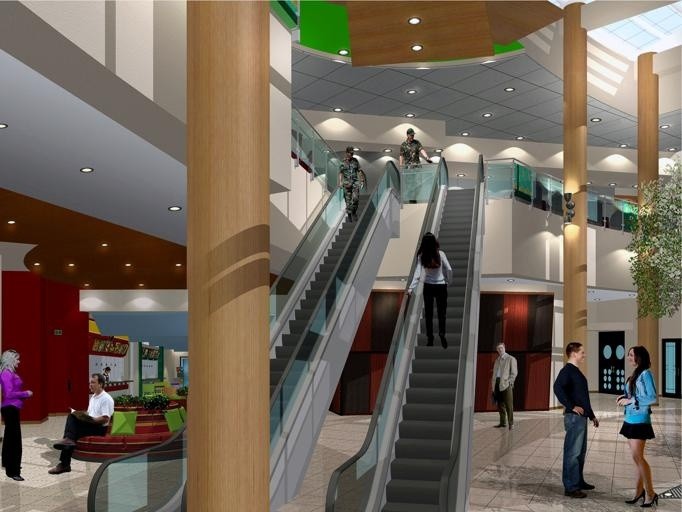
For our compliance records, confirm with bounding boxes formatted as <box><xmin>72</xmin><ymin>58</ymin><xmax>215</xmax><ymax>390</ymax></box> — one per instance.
<box><xmin>339</xmin><ymin>145</ymin><xmax>365</xmax><ymax>223</ymax></box>
<box><xmin>405</xmin><ymin>231</ymin><xmax>453</xmax><ymax>350</ymax></box>
<box><xmin>399</xmin><ymin>128</ymin><xmax>432</xmax><ymax>203</ymax></box>
<box><xmin>491</xmin><ymin>342</ymin><xmax>518</xmax><ymax>431</ymax></box>
<box><xmin>48</xmin><ymin>373</ymin><xmax>116</xmax><ymax>475</ymax></box>
<box><xmin>0</xmin><ymin>349</ymin><xmax>34</xmax><ymax>482</ymax></box>
<box><xmin>103</xmin><ymin>367</ymin><xmax>112</xmax><ymax>383</ymax></box>
<box><xmin>553</xmin><ymin>341</ymin><xmax>599</xmax><ymax>498</ymax></box>
<box><xmin>614</xmin><ymin>345</ymin><xmax>659</xmax><ymax>508</ymax></box>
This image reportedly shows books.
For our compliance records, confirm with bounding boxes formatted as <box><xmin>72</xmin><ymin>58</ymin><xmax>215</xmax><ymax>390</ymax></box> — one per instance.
<box><xmin>69</xmin><ymin>406</ymin><xmax>94</xmax><ymax>421</ymax></box>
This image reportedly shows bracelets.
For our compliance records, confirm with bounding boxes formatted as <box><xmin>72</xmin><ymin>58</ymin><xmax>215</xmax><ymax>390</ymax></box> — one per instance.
<box><xmin>426</xmin><ymin>156</ymin><xmax>429</xmax><ymax>161</ymax></box>
